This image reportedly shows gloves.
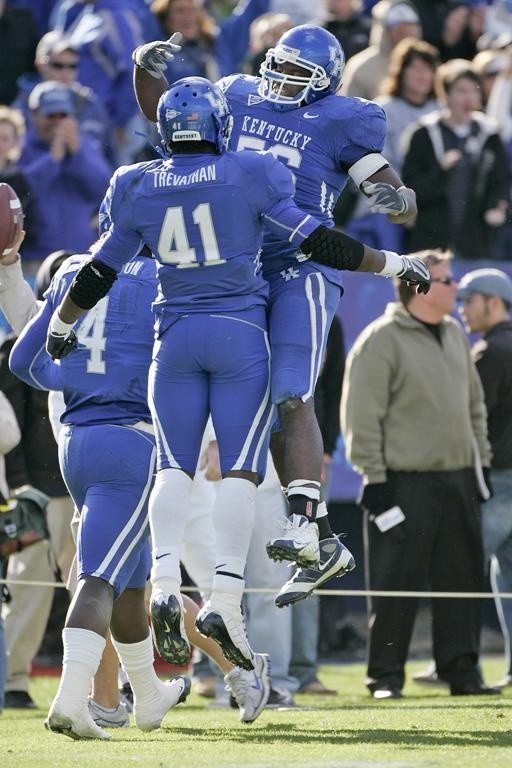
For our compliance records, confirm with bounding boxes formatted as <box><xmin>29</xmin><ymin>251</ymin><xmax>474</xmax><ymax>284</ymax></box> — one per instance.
<box><xmin>475</xmin><ymin>467</ymin><xmax>494</xmax><ymax>502</ymax></box>
<box><xmin>132</xmin><ymin>32</ymin><xmax>183</xmax><ymax>79</ymax></box>
<box><xmin>45</xmin><ymin>306</ymin><xmax>79</xmax><ymax>361</ymax></box>
<box><xmin>362</xmin><ymin>181</ymin><xmax>417</xmax><ymax>224</ymax></box>
<box><xmin>360</xmin><ymin>481</ymin><xmax>396</xmax><ymax>523</ymax></box>
<box><xmin>375</xmin><ymin>249</ymin><xmax>431</xmax><ymax>294</ymax></box>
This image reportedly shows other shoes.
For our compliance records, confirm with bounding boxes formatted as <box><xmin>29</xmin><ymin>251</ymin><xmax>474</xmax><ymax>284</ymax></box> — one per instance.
<box><xmin>6</xmin><ymin>690</ymin><xmax>36</xmax><ymax>709</ymax></box>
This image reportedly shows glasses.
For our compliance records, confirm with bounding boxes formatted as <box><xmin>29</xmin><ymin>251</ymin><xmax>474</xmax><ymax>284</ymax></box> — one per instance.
<box><xmin>431</xmin><ymin>276</ymin><xmax>451</xmax><ymax>285</ymax></box>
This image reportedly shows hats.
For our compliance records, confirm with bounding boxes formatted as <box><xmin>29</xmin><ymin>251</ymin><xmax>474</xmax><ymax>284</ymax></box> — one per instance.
<box><xmin>28</xmin><ymin>82</ymin><xmax>71</xmax><ymax>116</ymax></box>
<box><xmin>456</xmin><ymin>268</ymin><xmax>512</xmax><ymax>303</ymax></box>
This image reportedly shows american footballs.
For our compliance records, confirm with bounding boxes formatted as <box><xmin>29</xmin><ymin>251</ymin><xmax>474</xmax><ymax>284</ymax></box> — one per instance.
<box><xmin>1</xmin><ymin>181</ymin><xmax>22</xmax><ymax>258</ymax></box>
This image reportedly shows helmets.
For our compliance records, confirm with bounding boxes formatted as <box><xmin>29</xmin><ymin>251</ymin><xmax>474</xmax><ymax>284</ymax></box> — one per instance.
<box><xmin>157</xmin><ymin>76</ymin><xmax>234</xmax><ymax>155</ymax></box>
<box><xmin>258</xmin><ymin>24</ymin><xmax>346</xmax><ymax>112</ymax></box>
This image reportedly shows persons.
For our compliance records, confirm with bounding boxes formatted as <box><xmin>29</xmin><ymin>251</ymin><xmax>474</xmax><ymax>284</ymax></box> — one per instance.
<box><xmin>1</xmin><ymin>0</ymin><xmax>511</xmax><ymax>742</ymax></box>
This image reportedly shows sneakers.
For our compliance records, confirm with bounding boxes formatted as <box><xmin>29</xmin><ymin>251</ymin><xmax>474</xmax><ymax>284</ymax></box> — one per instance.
<box><xmin>86</xmin><ymin>695</ymin><xmax>130</xmax><ymax>728</ymax></box>
<box><xmin>195</xmin><ymin>599</ymin><xmax>256</xmax><ymax>671</ymax></box>
<box><xmin>275</xmin><ymin>532</ymin><xmax>355</xmax><ymax>608</ymax></box>
<box><xmin>373</xmin><ymin>685</ymin><xmax>403</xmax><ymax>699</ymax></box>
<box><xmin>225</xmin><ymin>653</ymin><xmax>270</xmax><ymax>724</ymax></box>
<box><xmin>265</xmin><ymin>514</ymin><xmax>320</xmax><ymax>569</ymax></box>
<box><xmin>133</xmin><ymin>675</ymin><xmax>191</xmax><ymax>733</ymax></box>
<box><xmin>413</xmin><ymin>662</ymin><xmax>512</xmax><ymax>695</ymax></box>
<box><xmin>230</xmin><ymin>684</ymin><xmax>295</xmax><ymax>708</ymax></box>
<box><xmin>43</xmin><ymin>696</ymin><xmax>109</xmax><ymax>737</ymax></box>
<box><xmin>147</xmin><ymin>589</ymin><xmax>191</xmax><ymax>665</ymax></box>
